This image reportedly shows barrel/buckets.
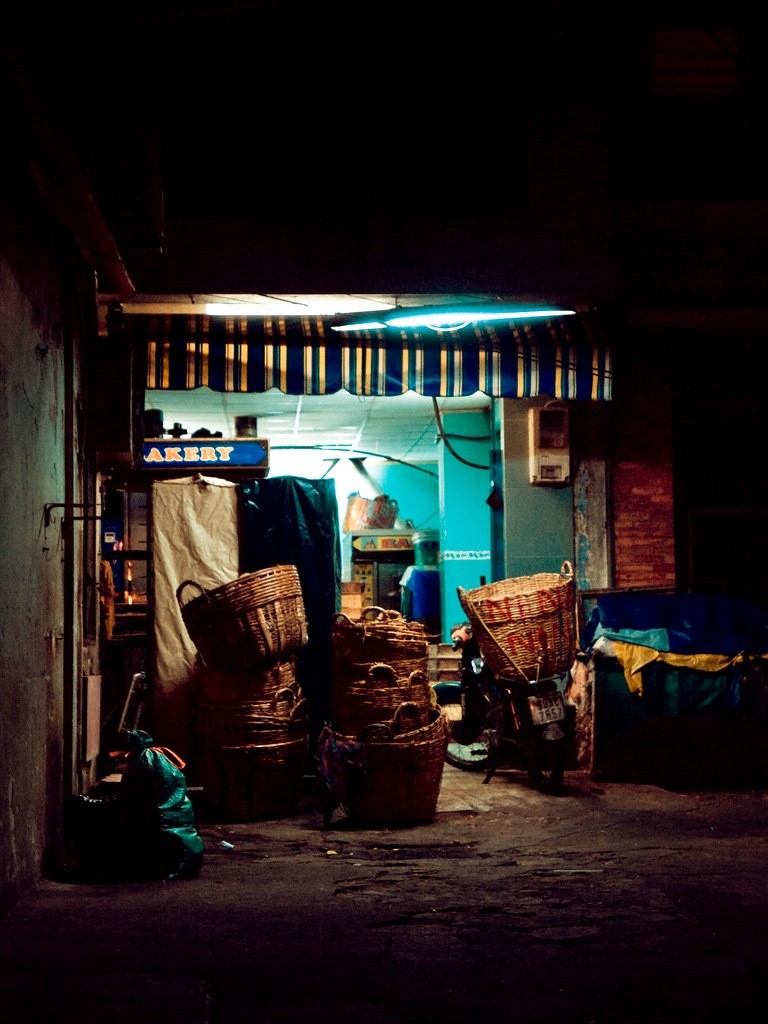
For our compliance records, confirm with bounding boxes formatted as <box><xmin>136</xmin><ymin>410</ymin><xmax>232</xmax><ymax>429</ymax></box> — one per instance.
<box><xmin>411</xmin><ymin>529</ymin><xmax>439</xmax><ymax>565</ymax></box>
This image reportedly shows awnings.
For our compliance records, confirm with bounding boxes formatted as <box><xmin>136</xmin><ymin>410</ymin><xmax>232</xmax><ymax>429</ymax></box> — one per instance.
<box><xmin>146</xmin><ymin>311</ymin><xmax>613</xmax><ymax>402</ymax></box>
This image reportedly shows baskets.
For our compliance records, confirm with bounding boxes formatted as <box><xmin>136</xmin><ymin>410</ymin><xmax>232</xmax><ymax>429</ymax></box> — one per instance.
<box><xmin>318</xmin><ymin>606</ymin><xmax>450</xmax><ymax>826</ymax></box>
<box><xmin>458</xmin><ymin>561</ymin><xmax>577</xmax><ymax>677</ymax></box>
<box><xmin>176</xmin><ymin>565</ymin><xmax>309</xmax><ymax>670</ymax></box>
<box><xmin>342</xmin><ymin>495</ymin><xmax>400</xmax><ymax>531</ymax></box>
<box><xmin>192</xmin><ymin>652</ymin><xmax>310</xmax><ymax>820</ymax></box>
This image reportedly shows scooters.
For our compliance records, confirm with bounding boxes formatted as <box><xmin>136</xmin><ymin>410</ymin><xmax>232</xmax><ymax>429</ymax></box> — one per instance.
<box><xmin>429</xmin><ymin>622</ymin><xmax>574</xmax><ymax>794</ymax></box>
